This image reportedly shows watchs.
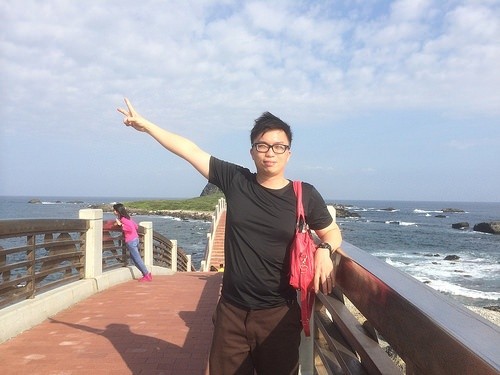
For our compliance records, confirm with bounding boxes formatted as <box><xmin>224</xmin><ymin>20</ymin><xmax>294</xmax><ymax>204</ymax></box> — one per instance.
<box><xmin>316</xmin><ymin>243</ymin><xmax>332</xmax><ymax>258</ymax></box>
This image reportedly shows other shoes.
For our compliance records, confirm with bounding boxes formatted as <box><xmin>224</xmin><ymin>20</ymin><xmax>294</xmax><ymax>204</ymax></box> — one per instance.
<box><xmin>138</xmin><ymin>273</ymin><xmax>152</xmax><ymax>281</ymax></box>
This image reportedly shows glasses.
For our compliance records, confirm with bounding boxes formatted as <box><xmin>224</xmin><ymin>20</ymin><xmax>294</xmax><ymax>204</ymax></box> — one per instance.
<box><xmin>252</xmin><ymin>142</ymin><xmax>291</xmax><ymax>154</ymax></box>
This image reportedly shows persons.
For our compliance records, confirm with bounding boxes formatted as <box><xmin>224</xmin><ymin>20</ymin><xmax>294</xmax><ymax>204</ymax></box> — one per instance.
<box><xmin>210</xmin><ymin>265</ymin><xmax>216</xmax><ymax>272</ymax></box>
<box><xmin>217</xmin><ymin>264</ymin><xmax>224</xmax><ymax>273</ymax></box>
<box><xmin>118</xmin><ymin>90</ymin><xmax>343</xmax><ymax>375</ymax></box>
<box><xmin>113</xmin><ymin>203</ymin><xmax>153</xmax><ymax>282</ymax></box>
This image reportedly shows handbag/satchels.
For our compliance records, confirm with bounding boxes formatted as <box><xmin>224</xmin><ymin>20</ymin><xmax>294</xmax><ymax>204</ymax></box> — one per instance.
<box><xmin>290</xmin><ymin>180</ymin><xmax>318</xmax><ymax>338</ymax></box>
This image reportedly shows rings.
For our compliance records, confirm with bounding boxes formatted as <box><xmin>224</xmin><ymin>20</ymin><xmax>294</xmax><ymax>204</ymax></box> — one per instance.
<box><xmin>326</xmin><ymin>276</ymin><xmax>331</xmax><ymax>279</ymax></box>
